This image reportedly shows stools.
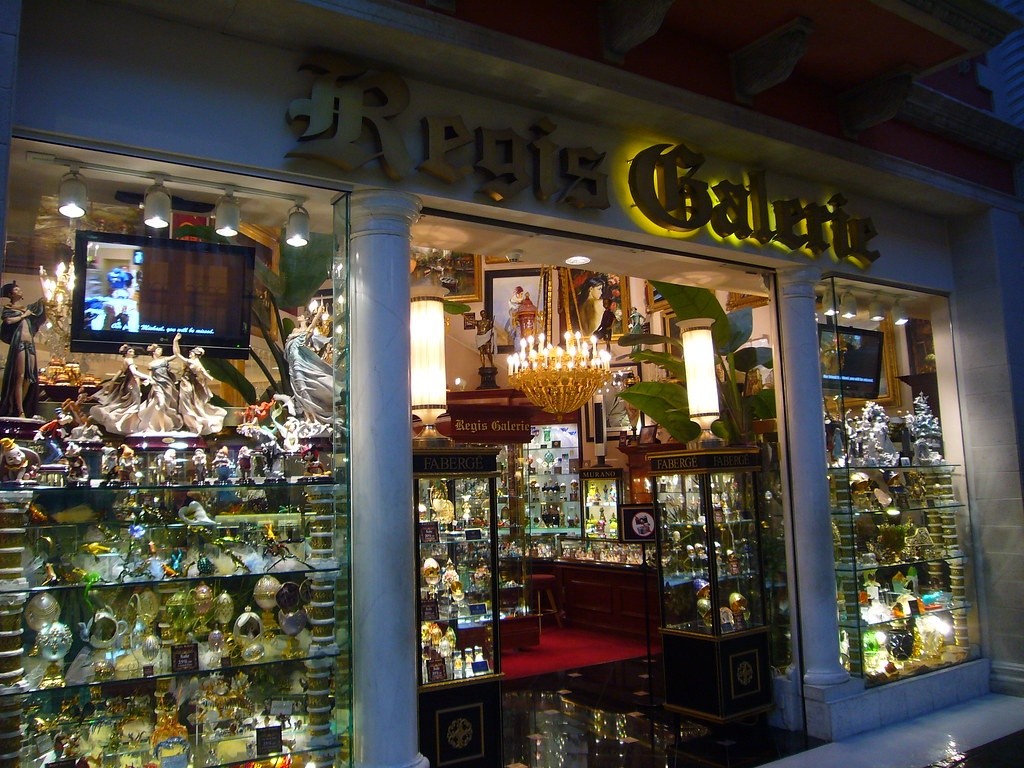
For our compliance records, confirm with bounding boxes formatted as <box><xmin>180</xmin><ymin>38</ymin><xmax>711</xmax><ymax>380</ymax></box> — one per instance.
<box><xmin>523</xmin><ymin>574</ymin><xmax>563</xmax><ymax>632</ymax></box>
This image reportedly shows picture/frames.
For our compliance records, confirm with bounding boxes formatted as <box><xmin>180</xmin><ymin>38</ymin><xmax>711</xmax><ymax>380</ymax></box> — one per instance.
<box><xmin>440</xmin><ymin>250</ymin><xmax>483</xmax><ymax>301</ymax></box>
<box><xmin>484</xmin><ymin>266</ymin><xmax>629</xmax><ymax>354</ymax></box>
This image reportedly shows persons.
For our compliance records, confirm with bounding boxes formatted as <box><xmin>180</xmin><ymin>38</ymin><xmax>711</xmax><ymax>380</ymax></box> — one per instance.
<box><xmin>504</xmin><ymin>286</ymin><xmax>524</xmax><ymax>332</ymax></box>
<box><xmin>89</xmin><ymin>344</ymin><xmax>150</xmax><ymax>435</ymax></box>
<box><xmin>832</xmin><ymin>408</ymin><xmax>928</xmax><ymax>512</ymax></box>
<box><xmin>139</xmin><ymin>343</ymin><xmax>183</xmax><ymax>432</ymax></box>
<box><xmin>284</xmin><ymin>305</ymin><xmax>333</xmax><ymax>425</ymax></box>
<box><xmin>686</xmin><ymin>492</ymin><xmax>750</xmax><ymax>632</ymax></box>
<box><xmin>0</xmin><ymin>283</ymin><xmax>45</xmax><ymax>418</ymax></box>
<box><xmin>462</xmin><ymin>310</ymin><xmax>495</xmax><ymax>368</ymax></box>
<box><xmin>628</xmin><ymin>307</ymin><xmax>646</xmax><ymax>352</ymax></box>
<box><xmin>0</xmin><ymin>405</ymin><xmax>331</xmax><ymax>485</ymax></box>
<box><xmin>575</xmin><ymin>276</ymin><xmax>605</xmax><ymax>335</ymax></box>
<box><xmin>593</xmin><ymin>299</ymin><xmax>618</xmax><ymax>354</ymax></box>
<box><xmin>173</xmin><ymin>333</ymin><xmax>228</xmax><ymax>435</ymax></box>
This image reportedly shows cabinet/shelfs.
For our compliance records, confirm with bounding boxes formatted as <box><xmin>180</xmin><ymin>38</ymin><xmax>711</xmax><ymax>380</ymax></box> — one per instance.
<box><xmin>1</xmin><ymin>487</ymin><xmax>352</xmax><ymax>768</ymax></box>
<box><xmin>647</xmin><ymin>455</ymin><xmax>773</xmax><ymax>721</ymax></box>
<box><xmin>411</xmin><ymin>451</ymin><xmax>503</xmax><ymax>768</ymax></box>
<box><xmin>831</xmin><ymin>466</ymin><xmax>974</xmax><ymax>688</ymax></box>
<box><xmin>518</xmin><ymin>424</ymin><xmax>580</xmax><ymax>534</ymax></box>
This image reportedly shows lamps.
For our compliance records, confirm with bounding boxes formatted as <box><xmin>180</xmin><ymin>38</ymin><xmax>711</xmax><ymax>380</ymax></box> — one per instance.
<box><xmin>820</xmin><ymin>283</ymin><xmax>911</xmax><ymax>325</ymax></box>
<box><xmin>507</xmin><ymin>265</ymin><xmax>613</xmax><ymax>421</ymax></box>
<box><xmin>60</xmin><ymin>171</ymin><xmax>308</xmax><ymax>247</ymax></box>
<box><xmin>40</xmin><ymin>258</ymin><xmax>83</xmax><ymax>385</ymax></box>
<box><xmin>676</xmin><ymin>318</ymin><xmax>726</xmax><ymax>448</ymax></box>
<box><xmin>410</xmin><ymin>288</ymin><xmax>454</xmax><ymax>446</ymax></box>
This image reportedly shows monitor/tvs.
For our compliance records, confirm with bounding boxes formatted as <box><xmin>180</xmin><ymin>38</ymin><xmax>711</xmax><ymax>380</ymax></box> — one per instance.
<box><xmin>817</xmin><ymin>323</ymin><xmax>885</xmax><ymax>399</ymax></box>
<box><xmin>70</xmin><ymin>229</ymin><xmax>255</xmax><ymax>360</ymax></box>
